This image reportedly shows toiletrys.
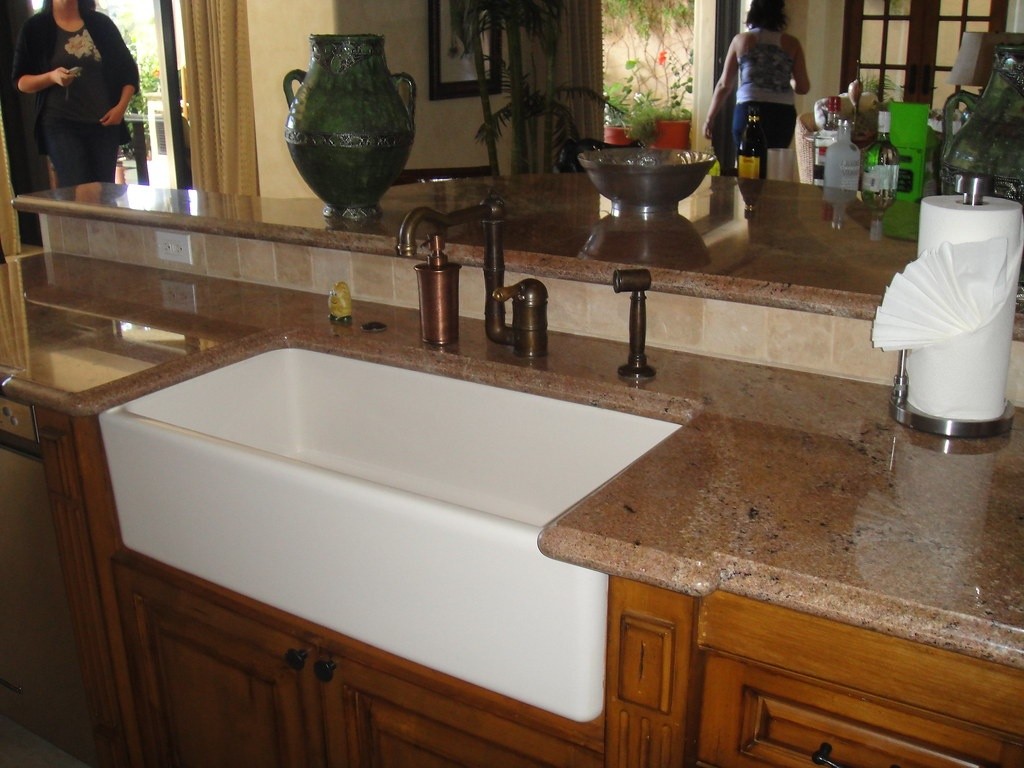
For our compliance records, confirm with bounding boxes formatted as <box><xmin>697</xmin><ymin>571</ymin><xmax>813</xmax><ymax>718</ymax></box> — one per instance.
<box><xmin>411</xmin><ymin>232</ymin><xmax>463</xmax><ymax>345</ymax></box>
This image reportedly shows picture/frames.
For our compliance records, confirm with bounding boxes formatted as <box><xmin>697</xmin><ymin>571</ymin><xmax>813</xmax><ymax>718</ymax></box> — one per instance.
<box><xmin>428</xmin><ymin>0</ymin><xmax>503</xmax><ymax>100</ymax></box>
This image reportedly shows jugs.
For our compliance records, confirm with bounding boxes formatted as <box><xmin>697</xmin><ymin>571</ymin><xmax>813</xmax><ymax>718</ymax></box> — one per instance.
<box><xmin>940</xmin><ymin>44</ymin><xmax>1024</xmax><ymax>203</ymax></box>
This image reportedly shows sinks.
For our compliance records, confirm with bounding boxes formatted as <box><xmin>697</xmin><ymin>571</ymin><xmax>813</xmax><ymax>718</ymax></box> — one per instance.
<box><xmin>97</xmin><ymin>345</ymin><xmax>686</xmax><ymax>724</ymax></box>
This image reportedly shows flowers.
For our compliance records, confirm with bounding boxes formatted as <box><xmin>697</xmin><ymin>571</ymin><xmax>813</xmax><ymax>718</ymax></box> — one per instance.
<box><xmin>625</xmin><ymin>51</ymin><xmax>698</xmax><ymax>143</ymax></box>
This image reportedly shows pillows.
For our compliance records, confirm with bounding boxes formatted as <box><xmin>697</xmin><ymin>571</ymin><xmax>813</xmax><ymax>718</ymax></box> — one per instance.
<box><xmin>814</xmin><ymin>91</ymin><xmax>877</xmax><ymax>130</ymax></box>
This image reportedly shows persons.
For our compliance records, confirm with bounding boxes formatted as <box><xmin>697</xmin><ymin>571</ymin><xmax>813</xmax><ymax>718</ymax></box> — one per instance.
<box><xmin>702</xmin><ymin>0</ymin><xmax>811</xmax><ymax>179</ymax></box>
<box><xmin>12</xmin><ymin>0</ymin><xmax>140</xmax><ymax>186</ymax></box>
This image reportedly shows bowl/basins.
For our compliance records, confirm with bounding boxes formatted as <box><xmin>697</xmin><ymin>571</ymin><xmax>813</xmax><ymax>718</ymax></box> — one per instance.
<box><xmin>577</xmin><ymin>146</ymin><xmax>718</xmax><ymax>207</ymax></box>
<box><xmin>577</xmin><ymin>203</ymin><xmax>712</xmax><ymax>274</ymax></box>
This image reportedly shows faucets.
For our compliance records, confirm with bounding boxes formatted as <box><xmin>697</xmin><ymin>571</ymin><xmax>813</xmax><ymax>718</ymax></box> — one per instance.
<box><xmin>392</xmin><ymin>185</ymin><xmax>511</xmax><ymax>345</ymax></box>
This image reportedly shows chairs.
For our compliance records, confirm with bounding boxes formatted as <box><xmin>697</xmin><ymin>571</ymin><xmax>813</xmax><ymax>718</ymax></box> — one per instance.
<box><xmin>794</xmin><ymin>92</ymin><xmax>880</xmax><ymax>185</ymax></box>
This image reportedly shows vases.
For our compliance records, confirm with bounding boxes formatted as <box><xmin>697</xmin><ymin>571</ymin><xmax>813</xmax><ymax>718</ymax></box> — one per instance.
<box><xmin>282</xmin><ymin>33</ymin><xmax>417</xmax><ymax>224</ymax></box>
<box><xmin>647</xmin><ymin>120</ymin><xmax>692</xmax><ymax>149</ymax></box>
<box><xmin>937</xmin><ymin>42</ymin><xmax>1024</xmax><ymax>285</ymax></box>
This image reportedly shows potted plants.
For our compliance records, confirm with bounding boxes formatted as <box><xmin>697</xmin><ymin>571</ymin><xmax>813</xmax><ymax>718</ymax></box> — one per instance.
<box><xmin>602</xmin><ymin>70</ymin><xmax>639</xmax><ymax>145</ymax></box>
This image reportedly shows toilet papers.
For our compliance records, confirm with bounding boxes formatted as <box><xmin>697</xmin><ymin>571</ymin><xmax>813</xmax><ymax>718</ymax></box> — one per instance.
<box><xmin>870</xmin><ymin>193</ymin><xmax>1024</xmax><ymax>425</ymax></box>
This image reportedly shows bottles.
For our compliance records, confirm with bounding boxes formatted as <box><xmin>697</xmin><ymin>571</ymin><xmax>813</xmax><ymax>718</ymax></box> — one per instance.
<box><xmin>860</xmin><ymin>111</ymin><xmax>901</xmax><ymax>210</ymax></box>
<box><xmin>821</xmin><ymin>119</ymin><xmax>861</xmax><ymax>203</ymax></box>
<box><xmin>737</xmin><ymin>104</ymin><xmax>767</xmax><ymax>179</ymax></box>
<box><xmin>812</xmin><ymin>96</ymin><xmax>842</xmax><ymax>187</ymax></box>
<box><xmin>282</xmin><ymin>33</ymin><xmax>417</xmax><ymax>228</ymax></box>
<box><xmin>737</xmin><ymin>178</ymin><xmax>763</xmax><ymax>219</ymax></box>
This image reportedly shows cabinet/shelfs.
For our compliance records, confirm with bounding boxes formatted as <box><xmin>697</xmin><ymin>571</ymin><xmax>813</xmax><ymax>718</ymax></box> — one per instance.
<box><xmin>112</xmin><ymin>559</ymin><xmax>1024</xmax><ymax>768</ymax></box>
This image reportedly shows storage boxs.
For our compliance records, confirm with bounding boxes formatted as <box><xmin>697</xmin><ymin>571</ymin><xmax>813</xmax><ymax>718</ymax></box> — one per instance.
<box><xmin>887</xmin><ymin>101</ymin><xmax>942</xmax><ymax>202</ymax></box>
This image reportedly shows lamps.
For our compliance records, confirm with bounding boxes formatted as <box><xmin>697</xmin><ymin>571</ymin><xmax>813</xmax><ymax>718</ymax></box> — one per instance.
<box><xmin>945</xmin><ymin>30</ymin><xmax>1024</xmax><ymax>94</ymax></box>
